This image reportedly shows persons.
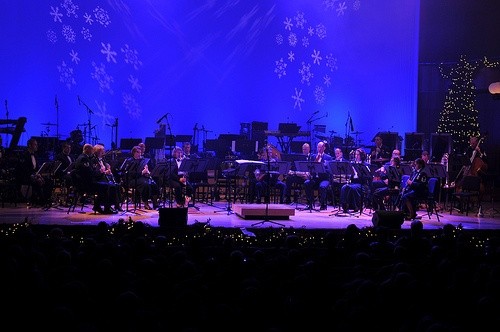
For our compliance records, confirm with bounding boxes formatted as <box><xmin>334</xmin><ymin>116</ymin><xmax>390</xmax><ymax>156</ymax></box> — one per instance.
<box><xmin>1</xmin><ymin>136</ymin><xmax>492</xmax><ymax>221</ymax></box>
<box><xmin>2</xmin><ymin>220</ymin><xmax>500</xmax><ymax>332</ymax></box>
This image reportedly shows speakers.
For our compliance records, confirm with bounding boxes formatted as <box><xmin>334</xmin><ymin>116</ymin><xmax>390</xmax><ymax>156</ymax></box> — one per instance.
<box><xmin>159</xmin><ymin>207</ymin><xmax>188</xmax><ymax>229</ymax></box>
<box><xmin>378</xmin><ymin>131</ymin><xmax>453</xmax><ymax>161</ymax></box>
<box><xmin>372</xmin><ymin>210</ymin><xmax>404</xmax><ymax>229</ymax></box>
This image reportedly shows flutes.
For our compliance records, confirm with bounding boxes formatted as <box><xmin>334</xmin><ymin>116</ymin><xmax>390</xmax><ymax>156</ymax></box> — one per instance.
<box><xmin>102</xmin><ymin>155</ymin><xmax>117</xmax><ymax>185</ymax></box>
<box><xmin>92</xmin><ymin>153</ymin><xmax>110</xmax><ymax>188</ymax></box>
<box><xmin>139</xmin><ymin>156</ymin><xmax>156</xmax><ymax>184</ymax></box>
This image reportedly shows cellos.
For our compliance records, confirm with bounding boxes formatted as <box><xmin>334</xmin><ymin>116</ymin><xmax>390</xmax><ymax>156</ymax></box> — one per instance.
<box><xmin>454</xmin><ymin>136</ymin><xmax>490</xmax><ymax>200</ymax></box>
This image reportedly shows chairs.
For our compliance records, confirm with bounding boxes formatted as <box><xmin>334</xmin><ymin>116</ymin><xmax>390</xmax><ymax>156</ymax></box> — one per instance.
<box><xmin>7</xmin><ymin>150</ymin><xmax>482</xmax><ymax>226</ymax></box>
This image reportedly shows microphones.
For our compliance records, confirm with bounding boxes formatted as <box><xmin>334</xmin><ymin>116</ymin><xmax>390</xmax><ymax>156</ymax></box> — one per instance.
<box><xmin>157</xmin><ymin>112</ymin><xmax>169</xmax><ymax>124</ymax></box>
<box><xmin>54</xmin><ymin>93</ymin><xmax>58</xmax><ymax>105</ymax></box>
<box><xmin>78</xmin><ymin>96</ymin><xmax>82</xmax><ymax>106</ymax></box>
<box><xmin>313</xmin><ymin>110</ymin><xmax>320</xmax><ymax>115</ymax></box>
<box><xmin>265</xmin><ymin>134</ymin><xmax>268</xmax><ymax>145</ymax></box>
<box><xmin>350</xmin><ymin>117</ymin><xmax>353</xmax><ymax>131</ymax></box>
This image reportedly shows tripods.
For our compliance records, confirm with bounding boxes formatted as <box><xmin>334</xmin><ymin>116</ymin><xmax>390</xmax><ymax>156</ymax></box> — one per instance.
<box><xmin>1</xmin><ymin>145</ymin><xmax>499</xmax><ymax>226</ymax></box>
